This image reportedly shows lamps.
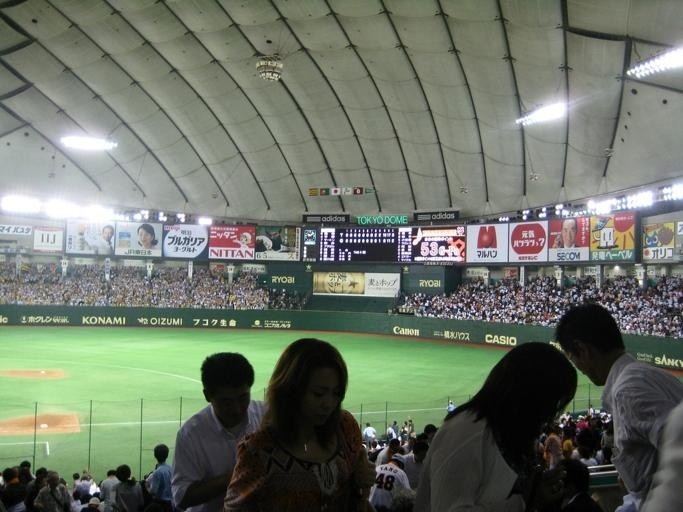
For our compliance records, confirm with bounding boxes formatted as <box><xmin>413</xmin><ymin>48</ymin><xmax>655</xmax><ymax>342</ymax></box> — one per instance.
<box><xmin>254</xmin><ymin>56</ymin><xmax>284</xmax><ymax>82</ymax></box>
<box><xmin>516</xmin><ymin>73</ymin><xmax>565</xmax><ymax>126</ymax></box>
<box><xmin>626</xmin><ymin>41</ymin><xmax>683</xmax><ymax>78</ymax></box>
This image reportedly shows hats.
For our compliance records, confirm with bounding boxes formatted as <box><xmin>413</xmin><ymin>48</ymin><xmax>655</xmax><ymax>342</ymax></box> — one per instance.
<box><xmin>392</xmin><ymin>453</ymin><xmax>405</xmax><ymax>469</ymax></box>
<box><xmin>89</xmin><ymin>497</ymin><xmax>101</xmax><ymax>506</ymax></box>
<box><xmin>563</xmin><ymin>441</ymin><xmax>575</xmax><ymax>451</ymax></box>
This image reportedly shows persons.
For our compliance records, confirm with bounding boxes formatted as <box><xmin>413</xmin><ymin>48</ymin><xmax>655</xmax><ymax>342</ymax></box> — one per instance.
<box><xmin>0</xmin><ymin>444</ymin><xmax>175</xmax><ymax>511</ymax></box>
<box><xmin>533</xmin><ymin>405</ymin><xmax>643</xmax><ymax>511</ymax></box>
<box><xmin>361</xmin><ymin>418</ymin><xmax>438</xmax><ymax>512</ymax></box>
<box><xmin>135</xmin><ymin>224</ymin><xmax>161</xmax><ymax>249</ymax></box>
<box><xmin>393</xmin><ymin>270</ymin><xmax>682</xmax><ymax>339</ymax></box>
<box><xmin>447</xmin><ymin>400</ymin><xmax>455</xmax><ymax>413</ymax></box>
<box><xmin>552</xmin><ymin>303</ymin><xmax>681</xmax><ymax>511</ymax></box>
<box><xmin>171</xmin><ymin>351</ymin><xmax>266</xmax><ymax>511</ymax></box>
<box><xmin>220</xmin><ymin>338</ymin><xmax>374</xmax><ymax>511</ymax></box>
<box><xmin>78</xmin><ymin>225</ymin><xmax>115</xmax><ymax>254</ymax></box>
<box><xmin>415</xmin><ymin>341</ymin><xmax>578</xmax><ymax>511</ymax></box>
<box><xmin>552</xmin><ymin>218</ymin><xmax>580</xmax><ymax>248</ymax></box>
<box><xmin>1</xmin><ymin>262</ymin><xmax>307</xmax><ymax>310</ymax></box>
